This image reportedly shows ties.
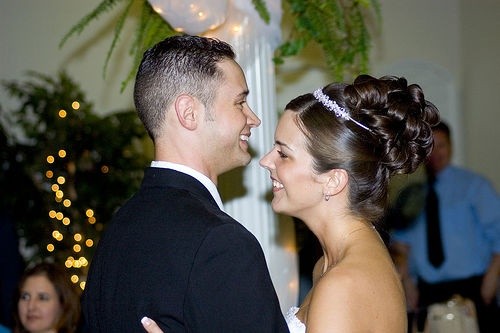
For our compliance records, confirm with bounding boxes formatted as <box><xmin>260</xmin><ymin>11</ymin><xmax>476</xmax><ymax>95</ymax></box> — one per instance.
<box><xmin>423</xmin><ymin>178</ymin><xmax>445</xmax><ymax>269</ymax></box>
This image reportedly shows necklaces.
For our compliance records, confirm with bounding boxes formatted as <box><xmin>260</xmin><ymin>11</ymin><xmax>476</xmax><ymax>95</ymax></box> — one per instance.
<box><xmin>319</xmin><ymin>225</ymin><xmax>375</xmax><ymax>278</ymax></box>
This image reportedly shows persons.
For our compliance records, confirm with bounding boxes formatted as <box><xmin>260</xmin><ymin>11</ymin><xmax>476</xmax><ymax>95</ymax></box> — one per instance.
<box><xmin>0</xmin><ymin>122</ymin><xmax>20</xmax><ymax>333</ymax></box>
<box><xmin>17</xmin><ymin>261</ymin><xmax>83</xmax><ymax>333</ymax></box>
<box><xmin>141</xmin><ymin>72</ymin><xmax>440</xmax><ymax>333</ymax></box>
<box><xmin>80</xmin><ymin>36</ymin><xmax>292</xmax><ymax>333</ymax></box>
<box><xmin>389</xmin><ymin>121</ymin><xmax>500</xmax><ymax>332</ymax></box>
